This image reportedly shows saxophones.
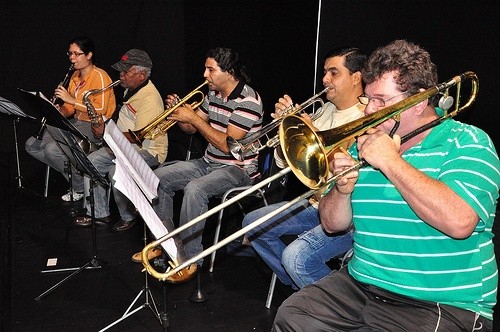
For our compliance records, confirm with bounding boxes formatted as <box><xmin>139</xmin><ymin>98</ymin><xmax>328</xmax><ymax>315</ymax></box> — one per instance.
<box><xmin>77</xmin><ymin>78</ymin><xmax>121</xmax><ymax>155</ymax></box>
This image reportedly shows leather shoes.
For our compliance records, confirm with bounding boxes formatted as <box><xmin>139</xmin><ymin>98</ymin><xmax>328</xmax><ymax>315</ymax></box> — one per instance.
<box><xmin>112</xmin><ymin>219</ymin><xmax>135</xmax><ymax>231</ymax></box>
<box><xmin>75</xmin><ymin>216</ymin><xmax>110</xmax><ymax>226</ymax></box>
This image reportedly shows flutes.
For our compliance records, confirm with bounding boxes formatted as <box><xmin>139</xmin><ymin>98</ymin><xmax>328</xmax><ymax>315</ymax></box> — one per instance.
<box><xmin>30</xmin><ymin>62</ymin><xmax>75</xmax><ymax>140</ymax></box>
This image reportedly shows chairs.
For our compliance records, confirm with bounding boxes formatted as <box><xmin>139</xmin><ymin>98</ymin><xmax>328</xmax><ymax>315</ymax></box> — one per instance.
<box><xmin>143</xmin><ymin>131</ymin><xmax>278</xmax><ymax>274</ymax></box>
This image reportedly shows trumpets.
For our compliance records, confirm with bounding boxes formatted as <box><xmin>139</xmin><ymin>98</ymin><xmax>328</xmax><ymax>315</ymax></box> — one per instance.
<box><xmin>128</xmin><ymin>79</ymin><xmax>210</xmax><ymax>148</ymax></box>
<box><xmin>227</xmin><ymin>87</ymin><xmax>330</xmax><ymax>162</ymax></box>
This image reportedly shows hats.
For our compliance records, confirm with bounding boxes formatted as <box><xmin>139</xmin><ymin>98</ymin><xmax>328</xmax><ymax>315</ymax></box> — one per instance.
<box><xmin>111</xmin><ymin>49</ymin><xmax>152</xmax><ymax>72</ymax></box>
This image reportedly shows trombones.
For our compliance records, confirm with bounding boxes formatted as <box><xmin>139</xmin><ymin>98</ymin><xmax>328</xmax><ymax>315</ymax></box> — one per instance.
<box><xmin>141</xmin><ymin>71</ymin><xmax>479</xmax><ymax>281</ymax></box>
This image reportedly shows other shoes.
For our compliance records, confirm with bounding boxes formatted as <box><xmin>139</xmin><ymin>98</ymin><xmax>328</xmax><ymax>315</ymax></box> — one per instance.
<box><xmin>61</xmin><ymin>190</ymin><xmax>84</xmax><ymax>201</ymax></box>
<box><xmin>166</xmin><ymin>263</ymin><xmax>197</xmax><ymax>281</ymax></box>
<box><xmin>132</xmin><ymin>246</ymin><xmax>163</xmax><ymax>263</ymax></box>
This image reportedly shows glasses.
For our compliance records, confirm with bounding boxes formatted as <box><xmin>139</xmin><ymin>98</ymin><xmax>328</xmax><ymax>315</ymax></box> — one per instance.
<box><xmin>357</xmin><ymin>89</ymin><xmax>412</xmax><ymax>107</ymax></box>
<box><xmin>67</xmin><ymin>51</ymin><xmax>87</xmax><ymax>57</ymax></box>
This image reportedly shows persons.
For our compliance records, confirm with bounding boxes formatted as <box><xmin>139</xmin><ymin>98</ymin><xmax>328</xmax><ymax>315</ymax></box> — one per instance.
<box><xmin>24</xmin><ymin>37</ymin><xmax>117</xmax><ymax>201</ymax></box>
<box><xmin>241</xmin><ymin>47</ymin><xmax>369</xmax><ymax>291</ymax></box>
<box><xmin>73</xmin><ymin>50</ymin><xmax>169</xmax><ymax>233</ymax></box>
<box><xmin>130</xmin><ymin>46</ymin><xmax>264</xmax><ymax>284</ymax></box>
<box><xmin>271</xmin><ymin>39</ymin><xmax>500</xmax><ymax>331</ymax></box>
